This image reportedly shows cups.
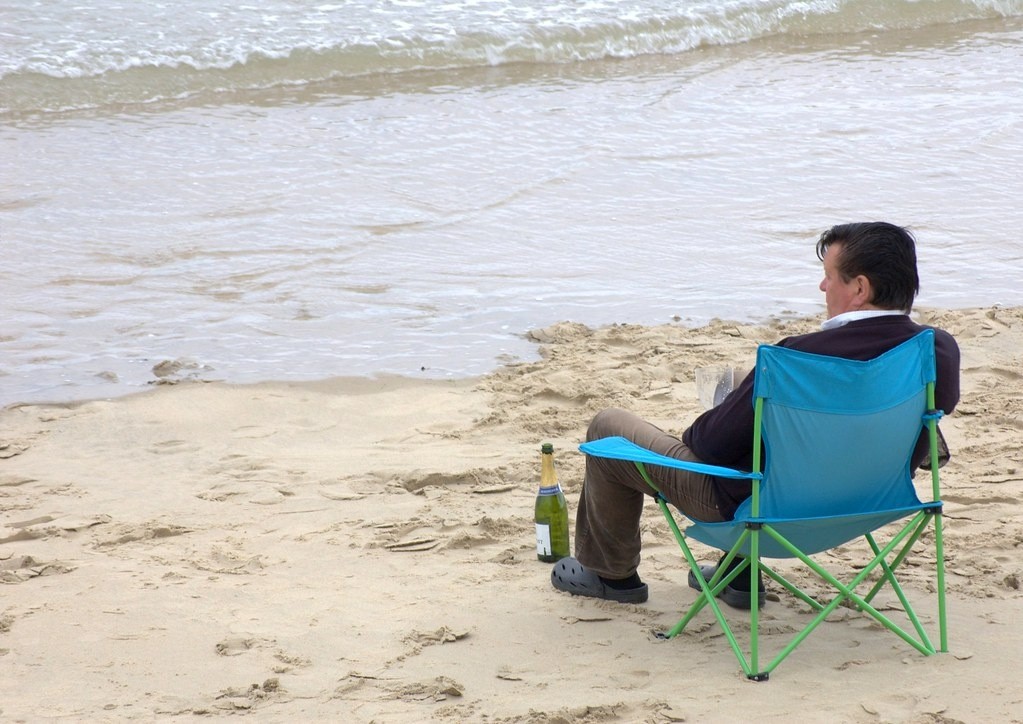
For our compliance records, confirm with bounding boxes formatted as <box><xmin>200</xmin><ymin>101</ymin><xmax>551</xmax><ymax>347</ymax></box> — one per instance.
<box><xmin>694</xmin><ymin>365</ymin><xmax>734</xmax><ymax>411</ymax></box>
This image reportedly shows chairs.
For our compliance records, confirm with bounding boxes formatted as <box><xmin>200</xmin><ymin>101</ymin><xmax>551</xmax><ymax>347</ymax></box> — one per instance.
<box><xmin>578</xmin><ymin>329</ymin><xmax>949</xmax><ymax>681</ymax></box>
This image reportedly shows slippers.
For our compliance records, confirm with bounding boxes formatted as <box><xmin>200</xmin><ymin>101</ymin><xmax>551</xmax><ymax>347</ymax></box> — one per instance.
<box><xmin>689</xmin><ymin>561</ymin><xmax>766</xmax><ymax>608</ymax></box>
<box><xmin>551</xmin><ymin>556</ymin><xmax>649</xmax><ymax>604</ymax></box>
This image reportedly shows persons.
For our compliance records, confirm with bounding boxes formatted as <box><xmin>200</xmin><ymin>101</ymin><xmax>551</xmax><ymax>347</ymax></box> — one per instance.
<box><xmin>550</xmin><ymin>221</ymin><xmax>961</xmax><ymax>607</ymax></box>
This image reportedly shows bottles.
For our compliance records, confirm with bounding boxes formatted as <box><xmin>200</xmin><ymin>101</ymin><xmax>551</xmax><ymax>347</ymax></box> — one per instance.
<box><xmin>534</xmin><ymin>443</ymin><xmax>570</xmax><ymax>563</ymax></box>
